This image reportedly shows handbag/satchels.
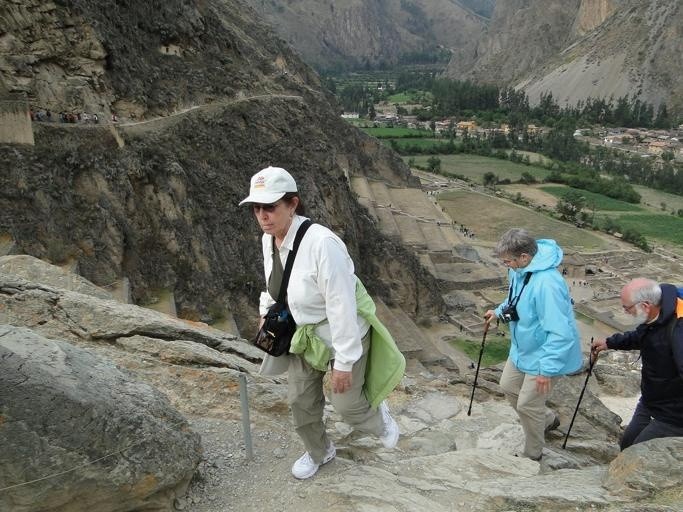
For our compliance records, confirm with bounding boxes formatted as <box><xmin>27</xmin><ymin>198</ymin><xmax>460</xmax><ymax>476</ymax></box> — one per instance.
<box><xmin>253</xmin><ymin>302</ymin><xmax>296</xmax><ymax>357</ymax></box>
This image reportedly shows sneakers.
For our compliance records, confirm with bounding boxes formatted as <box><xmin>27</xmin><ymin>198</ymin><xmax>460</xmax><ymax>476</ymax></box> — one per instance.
<box><xmin>545</xmin><ymin>416</ymin><xmax>559</xmax><ymax>435</ymax></box>
<box><xmin>376</xmin><ymin>401</ymin><xmax>399</xmax><ymax>450</ymax></box>
<box><xmin>292</xmin><ymin>438</ymin><xmax>336</xmax><ymax>479</ymax></box>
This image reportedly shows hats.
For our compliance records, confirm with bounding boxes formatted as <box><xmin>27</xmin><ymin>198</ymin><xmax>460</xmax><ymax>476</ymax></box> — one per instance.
<box><xmin>238</xmin><ymin>165</ymin><xmax>298</xmax><ymax>206</ymax></box>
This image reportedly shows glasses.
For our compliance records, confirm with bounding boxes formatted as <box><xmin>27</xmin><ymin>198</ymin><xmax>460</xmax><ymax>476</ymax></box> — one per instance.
<box><xmin>252</xmin><ymin>203</ymin><xmax>282</xmax><ymax>213</ymax></box>
<box><xmin>623</xmin><ymin>301</ymin><xmax>640</xmax><ymax>313</ymax></box>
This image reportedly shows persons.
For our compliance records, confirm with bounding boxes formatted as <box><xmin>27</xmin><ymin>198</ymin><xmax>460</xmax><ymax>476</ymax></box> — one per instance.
<box><xmin>588</xmin><ymin>276</ymin><xmax>682</xmax><ymax>452</ymax></box>
<box><xmin>239</xmin><ymin>164</ymin><xmax>399</xmax><ymax>480</ymax></box>
<box><xmin>480</xmin><ymin>227</ymin><xmax>585</xmax><ymax>461</ymax></box>
<box><xmin>30</xmin><ymin>106</ymin><xmax>145</xmax><ymax>124</ymax></box>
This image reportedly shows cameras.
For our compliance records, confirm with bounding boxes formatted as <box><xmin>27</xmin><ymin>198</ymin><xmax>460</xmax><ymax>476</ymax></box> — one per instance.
<box><xmin>498</xmin><ymin>304</ymin><xmax>520</xmax><ymax>323</ymax></box>
<box><xmin>253</xmin><ymin>312</ymin><xmax>294</xmax><ymax>357</ymax></box>
